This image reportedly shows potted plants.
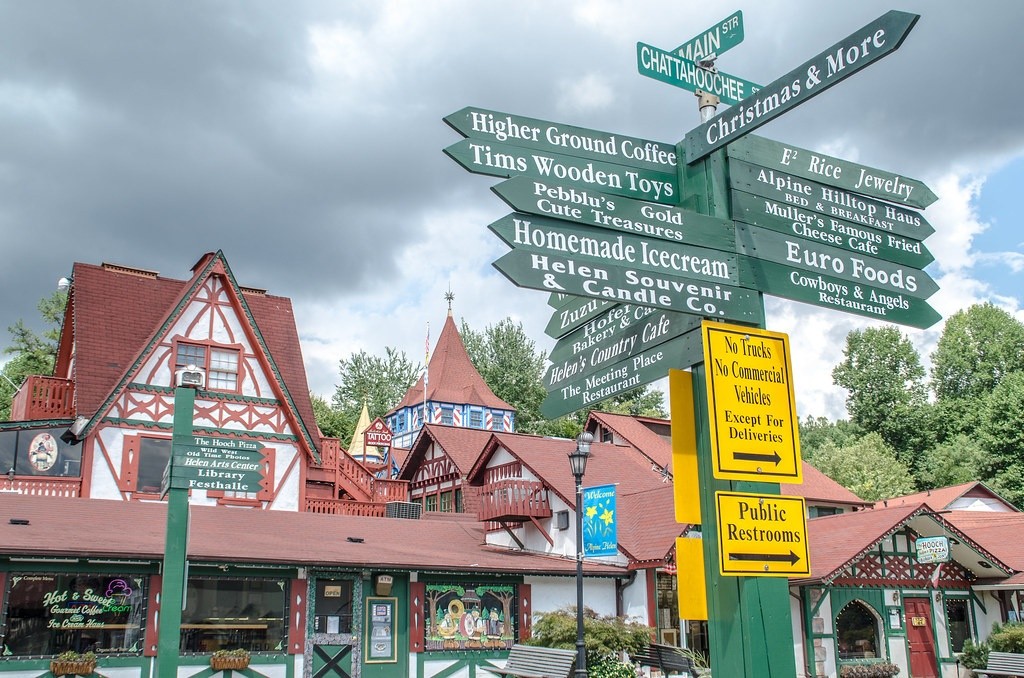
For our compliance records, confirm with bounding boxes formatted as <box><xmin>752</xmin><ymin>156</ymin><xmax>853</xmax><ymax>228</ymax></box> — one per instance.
<box><xmin>209</xmin><ymin>648</ymin><xmax>251</xmax><ymax>671</ymax></box>
<box><xmin>48</xmin><ymin>649</ymin><xmax>98</xmax><ymax>675</ymax></box>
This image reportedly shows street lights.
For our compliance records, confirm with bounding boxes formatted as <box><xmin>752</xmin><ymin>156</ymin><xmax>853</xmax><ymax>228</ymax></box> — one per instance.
<box><xmin>567</xmin><ymin>442</ymin><xmax>596</xmax><ymax>678</ymax></box>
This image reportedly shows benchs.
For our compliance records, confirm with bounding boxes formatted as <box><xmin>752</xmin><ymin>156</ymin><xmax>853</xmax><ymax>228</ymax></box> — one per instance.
<box><xmin>479</xmin><ymin>644</ymin><xmax>578</xmax><ymax>678</ymax></box>
<box><xmin>628</xmin><ymin>643</ymin><xmax>711</xmax><ymax>678</ymax></box>
<box><xmin>971</xmin><ymin>651</ymin><xmax>1024</xmax><ymax>678</ymax></box>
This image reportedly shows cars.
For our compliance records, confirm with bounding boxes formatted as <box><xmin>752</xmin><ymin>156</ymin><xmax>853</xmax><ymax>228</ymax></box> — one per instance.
<box><xmin>9</xmin><ymin>618</ymin><xmax>37</xmax><ymax>640</ymax></box>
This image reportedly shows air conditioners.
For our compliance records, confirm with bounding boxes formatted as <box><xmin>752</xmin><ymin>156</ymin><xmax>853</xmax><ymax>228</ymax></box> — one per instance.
<box><xmin>174</xmin><ymin>370</ymin><xmax>204</xmax><ymax>388</ymax></box>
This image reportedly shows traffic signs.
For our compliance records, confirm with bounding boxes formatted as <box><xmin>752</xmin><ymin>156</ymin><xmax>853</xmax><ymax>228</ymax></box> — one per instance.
<box><xmin>160</xmin><ymin>435</ymin><xmax>267</xmax><ymax>501</ymax></box>
<box><xmin>443</xmin><ymin>3</ymin><xmax>942</xmax><ymax>422</ymax></box>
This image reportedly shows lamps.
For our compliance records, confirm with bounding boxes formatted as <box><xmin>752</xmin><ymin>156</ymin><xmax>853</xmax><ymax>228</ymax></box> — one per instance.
<box><xmin>57</xmin><ymin>277</ymin><xmax>73</xmax><ymax>290</ymax></box>
